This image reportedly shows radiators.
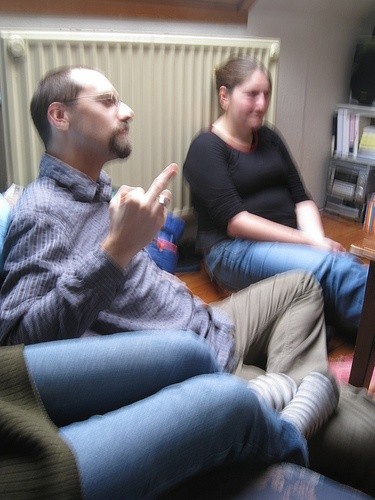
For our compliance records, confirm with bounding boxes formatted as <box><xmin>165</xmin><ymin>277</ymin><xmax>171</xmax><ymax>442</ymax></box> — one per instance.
<box><xmin>0</xmin><ymin>31</ymin><xmax>281</xmax><ymax>217</ymax></box>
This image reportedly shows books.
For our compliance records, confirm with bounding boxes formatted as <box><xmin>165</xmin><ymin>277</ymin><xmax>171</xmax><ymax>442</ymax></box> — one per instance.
<box><xmin>331</xmin><ymin>107</ymin><xmax>375</xmax><ymax>161</ymax></box>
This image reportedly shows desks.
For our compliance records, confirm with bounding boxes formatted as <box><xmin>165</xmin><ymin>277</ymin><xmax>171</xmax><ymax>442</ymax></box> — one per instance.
<box><xmin>348</xmin><ymin>234</ymin><xmax>375</xmax><ymax>390</ymax></box>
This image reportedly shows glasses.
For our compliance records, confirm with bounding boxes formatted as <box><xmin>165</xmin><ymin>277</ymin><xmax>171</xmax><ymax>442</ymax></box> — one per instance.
<box><xmin>60</xmin><ymin>92</ymin><xmax>120</xmax><ymax>106</ymax></box>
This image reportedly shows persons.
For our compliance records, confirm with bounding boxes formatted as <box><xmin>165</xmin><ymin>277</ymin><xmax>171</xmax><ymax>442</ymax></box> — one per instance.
<box><xmin>185</xmin><ymin>56</ymin><xmax>368</xmax><ymax>332</ymax></box>
<box><xmin>0</xmin><ymin>324</ymin><xmax>340</xmax><ymax>500</ymax></box>
<box><xmin>1</xmin><ymin>65</ymin><xmax>375</xmax><ymax>487</ymax></box>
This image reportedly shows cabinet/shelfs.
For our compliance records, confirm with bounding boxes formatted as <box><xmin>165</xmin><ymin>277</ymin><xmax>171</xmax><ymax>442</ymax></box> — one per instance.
<box><xmin>331</xmin><ymin>103</ymin><xmax>375</xmax><ymax>165</ymax></box>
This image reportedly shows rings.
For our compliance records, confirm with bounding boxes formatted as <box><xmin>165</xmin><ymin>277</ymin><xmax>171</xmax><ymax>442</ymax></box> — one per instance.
<box><xmin>156</xmin><ymin>191</ymin><xmax>171</xmax><ymax>210</ymax></box>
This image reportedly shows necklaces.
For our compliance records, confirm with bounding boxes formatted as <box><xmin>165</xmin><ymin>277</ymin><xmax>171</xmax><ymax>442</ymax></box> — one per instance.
<box><xmin>210</xmin><ymin>123</ymin><xmax>252</xmax><ymax>147</ymax></box>
